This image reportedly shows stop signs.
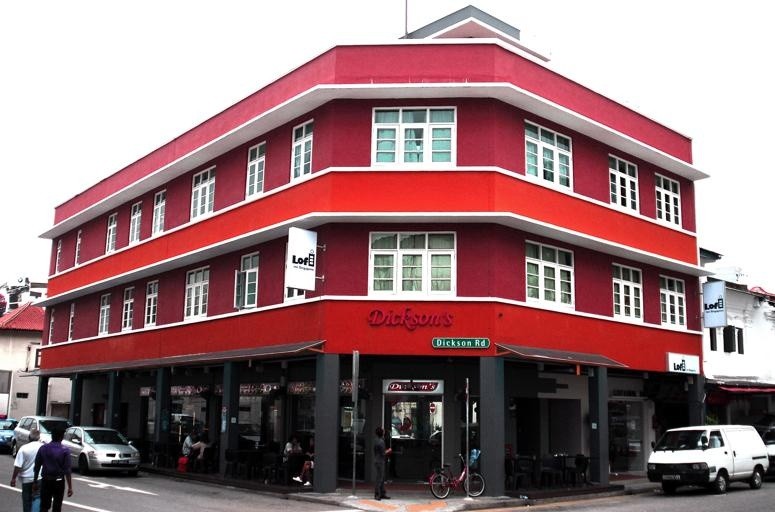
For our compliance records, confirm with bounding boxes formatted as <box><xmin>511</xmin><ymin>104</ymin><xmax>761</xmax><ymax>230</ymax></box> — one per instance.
<box><xmin>430</xmin><ymin>404</ymin><xmax>435</xmax><ymax>412</ymax></box>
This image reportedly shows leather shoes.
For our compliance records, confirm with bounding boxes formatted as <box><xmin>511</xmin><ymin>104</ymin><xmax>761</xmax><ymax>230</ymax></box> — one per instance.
<box><xmin>375</xmin><ymin>495</ymin><xmax>391</xmax><ymax>500</ymax></box>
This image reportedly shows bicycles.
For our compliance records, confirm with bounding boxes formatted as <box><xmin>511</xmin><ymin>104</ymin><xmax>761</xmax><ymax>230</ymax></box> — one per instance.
<box><xmin>428</xmin><ymin>448</ymin><xmax>486</xmax><ymax>499</ymax></box>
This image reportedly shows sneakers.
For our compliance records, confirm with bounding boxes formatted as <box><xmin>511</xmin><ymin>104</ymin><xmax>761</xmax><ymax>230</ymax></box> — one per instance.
<box><xmin>292</xmin><ymin>476</ymin><xmax>302</xmax><ymax>483</ymax></box>
<box><xmin>304</xmin><ymin>482</ymin><xmax>312</xmax><ymax>486</ymax></box>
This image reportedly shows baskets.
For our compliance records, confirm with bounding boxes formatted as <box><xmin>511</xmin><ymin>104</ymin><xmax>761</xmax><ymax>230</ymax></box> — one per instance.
<box><xmin>468</xmin><ymin>459</ymin><xmax>478</xmax><ymax>468</ymax></box>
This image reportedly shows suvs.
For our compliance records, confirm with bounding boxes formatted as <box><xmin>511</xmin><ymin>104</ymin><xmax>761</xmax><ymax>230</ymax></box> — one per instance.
<box><xmin>238</xmin><ymin>419</ymin><xmax>261</xmax><ymax>451</ymax></box>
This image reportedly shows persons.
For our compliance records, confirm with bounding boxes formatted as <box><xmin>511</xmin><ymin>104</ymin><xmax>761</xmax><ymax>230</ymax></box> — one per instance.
<box><xmin>291</xmin><ymin>435</ymin><xmax>314</xmax><ymax>487</ymax></box>
<box><xmin>371</xmin><ymin>426</ymin><xmax>394</xmax><ymax>500</ymax></box>
<box><xmin>282</xmin><ymin>434</ymin><xmax>303</xmax><ymax>463</ymax></box>
<box><xmin>179</xmin><ymin>431</ymin><xmax>200</xmax><ymax>461</ymax></box>
<box><xmin>430</xmin><ymin>425</ymin><xmax>441</xmax><ymax>438</ymax></box>
<box><xmin>9</xmin><ymin>427</ymin><xmax>44</xmax><ymax>512</ymax></box>
<box><xmin>31</xmin><ymin>424</ymin><xmax>73</xmax><ymax>511</ymax></box>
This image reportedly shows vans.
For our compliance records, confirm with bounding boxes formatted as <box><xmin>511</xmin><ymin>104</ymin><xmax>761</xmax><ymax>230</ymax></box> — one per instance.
<box><xmin>647</xmin><ymin>424</ymin><xmax>770</xmax><ymax>493</ymax></box>
<box><xmin>756</xmin><ymin>425</ymin><xmax>775</xmax><ymax>477</ymax></box>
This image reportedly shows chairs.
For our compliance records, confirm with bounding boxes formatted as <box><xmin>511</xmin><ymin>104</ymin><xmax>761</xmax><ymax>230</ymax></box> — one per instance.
<box><xmin>223</xmin><ymin>440</ymin><xmax>314</xmax><ymax>487</ymax></box>
<box><xmin>505</xmin><ymin>452</ymin><xmax>589</xmax><ymax>490</ymax></box>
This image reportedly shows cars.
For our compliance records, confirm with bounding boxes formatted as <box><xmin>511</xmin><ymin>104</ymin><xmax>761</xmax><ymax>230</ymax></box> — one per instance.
<box><xmin>294</xmin><ymin>429</ymin><xmax>315</xmax><ymax>439</ymax></box>
<box><xmin>166</xmin><ymin>412</ymin><xmax>195</xmax><ymax>430</ymax></box>
<box><xmin>0</xmin><ymin>412</ymin><xmax>140</xmax><ymax>476</ymax></box>
<box><xmin>350</xmin><ymin>418</ymin><xmax>480</xmax><ymax>450</ymax></box>
